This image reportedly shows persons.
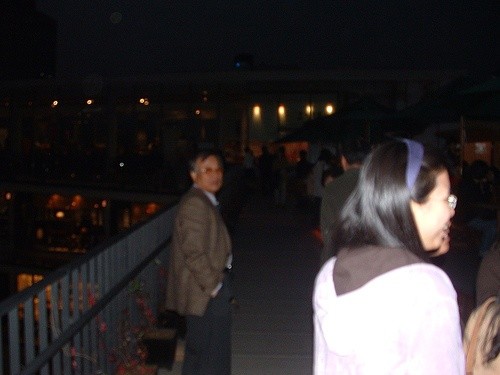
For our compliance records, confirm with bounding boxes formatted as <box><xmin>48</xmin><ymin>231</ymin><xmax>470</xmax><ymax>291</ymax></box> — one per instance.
<box><xmin>0</xmin><ymin>131</ymin><xmax>500</xmax><ymax>375</ymax></box>
<box><xmin>310</xmin><ymin>137</ymin><xmax>466</xmax><ymax>375</ymax></box>
<box><xmin>165</xmin><ymin>142</ymin><xmax>241</xmax><ymax>375</ymax></box>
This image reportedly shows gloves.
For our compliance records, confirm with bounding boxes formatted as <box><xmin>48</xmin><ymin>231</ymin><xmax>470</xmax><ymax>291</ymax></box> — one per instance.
<box><xmin>218</xmin><ymin>286</ymin><xmax>245</xmax><ymax>310</ymax></box>
